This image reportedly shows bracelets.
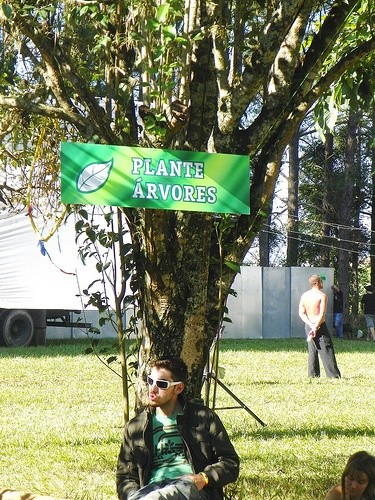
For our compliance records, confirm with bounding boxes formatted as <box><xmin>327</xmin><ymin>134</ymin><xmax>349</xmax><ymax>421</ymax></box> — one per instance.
<box><xmin>197</xmin><ymin>471</ymin><xmax>209</xmax><ymax>484</ymax></box>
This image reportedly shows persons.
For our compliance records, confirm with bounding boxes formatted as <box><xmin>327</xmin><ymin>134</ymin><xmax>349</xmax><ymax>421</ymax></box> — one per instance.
<box><xmin>115</xmin><ymin>356</ymin><xmax>239</xmax><ymax>500</ymax></box>
<box><xmin>300</xmin><ymin>274</ymin><xmax>375</xmax><ymax>379</ymax></box>
<box><xmin>324</xmin><ymin>450</ymin><xmax>375</xmax><ymax>500</ymax></box>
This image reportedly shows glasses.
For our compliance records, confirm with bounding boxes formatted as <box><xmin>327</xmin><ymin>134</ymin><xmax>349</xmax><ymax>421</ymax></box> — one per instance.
<box><xmin>147</xmin><ymin>375</ymin><xmax>182</xmax><ymax>389</ymax></box>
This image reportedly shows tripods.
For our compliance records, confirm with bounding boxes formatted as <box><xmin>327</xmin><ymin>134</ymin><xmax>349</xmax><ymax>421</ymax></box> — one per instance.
<box><xmin>199</xmin><ymin>352</ymin><xmax>267</xmax><ymax>427</ymax></box>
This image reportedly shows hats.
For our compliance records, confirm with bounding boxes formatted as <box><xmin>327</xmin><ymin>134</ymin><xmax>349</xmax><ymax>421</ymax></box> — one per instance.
<box><xmin>364</xmin><ymin>286</ymin><xmax>372</xmax><ymax>291</ymax></box>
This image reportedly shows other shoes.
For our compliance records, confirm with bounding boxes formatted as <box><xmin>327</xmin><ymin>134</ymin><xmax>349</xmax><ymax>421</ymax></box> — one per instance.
<box><xmin>365</xmin><ymin>338</ymin><xmax>369</xmax><ymax>341</ymax></box>
